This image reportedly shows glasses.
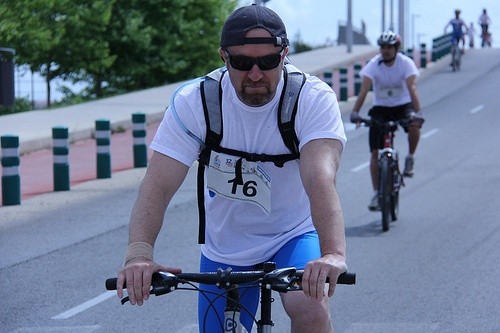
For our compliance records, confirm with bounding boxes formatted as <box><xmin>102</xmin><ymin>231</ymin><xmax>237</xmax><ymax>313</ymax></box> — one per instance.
<box><xmin>221</xmin><ymin>47</ymin><xmax>284</xmax><ymax>71</ymax></box>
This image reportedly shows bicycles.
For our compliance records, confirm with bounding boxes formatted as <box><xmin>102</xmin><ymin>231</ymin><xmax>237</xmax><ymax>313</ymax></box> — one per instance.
<box><xmin>105</xmin><ymin>261</ymin><xmax>356</xmax><ymax>333</ymax></box>
<box><xmin>446</xmin><ymin>31</ymin><xmax>466</xmax><ymax>72</ymax></box>
<box><xmin>352</xmin><ymin>115</ymin><xmax>414</xmax><ymax>231</ymax></box>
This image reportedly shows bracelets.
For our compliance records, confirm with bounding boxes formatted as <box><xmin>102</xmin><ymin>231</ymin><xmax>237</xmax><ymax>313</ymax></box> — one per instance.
<box><xmin>124</xmin><ymin>241</ymin><xmax>154</xmax><ymax>266</ymax></box>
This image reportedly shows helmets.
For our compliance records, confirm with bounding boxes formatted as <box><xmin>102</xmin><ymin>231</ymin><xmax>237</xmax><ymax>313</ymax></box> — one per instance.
<box><xmin>377</xmin><ymin>31</ymin><xmax>401</xmax><ymax>47</ymax></box>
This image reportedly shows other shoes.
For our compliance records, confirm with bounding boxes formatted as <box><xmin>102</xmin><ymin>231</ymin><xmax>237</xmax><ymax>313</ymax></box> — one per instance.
<box><xmin>403</xmin><ymin>155</ymin><xmax>414</xmax><ymax>177</ymax></box>
<box><xmin>369</xmin><ymin>194</ymin><xmax>380</xmax><ymax>209</ymax></box>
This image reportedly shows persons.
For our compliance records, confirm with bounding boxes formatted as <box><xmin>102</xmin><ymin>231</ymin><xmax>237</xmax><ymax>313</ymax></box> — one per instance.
<box><xmin>350</xmin><ymin>31</ymin><xmax>426</xmax><ymax>211</ymax></box>
<box><xmin>469</xmin><ymin>8</ymin><xmax>492</xmax><ymax>47</ymax></box>
<box><xmin>116</xmin><ymin>3</ymin><xmax>348</xmax><ymax>333</ymax></box>
<box><xmin>444</xmin><ymin>8</ymin><xmax>469</xmax><ymax>66</ymax></box>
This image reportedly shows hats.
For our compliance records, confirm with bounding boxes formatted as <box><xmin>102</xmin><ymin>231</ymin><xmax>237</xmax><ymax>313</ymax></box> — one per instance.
<box><xmin>220</xmin><ymin>3</ymin><xmax>290</xmax><ymax>48</ymax></box>
